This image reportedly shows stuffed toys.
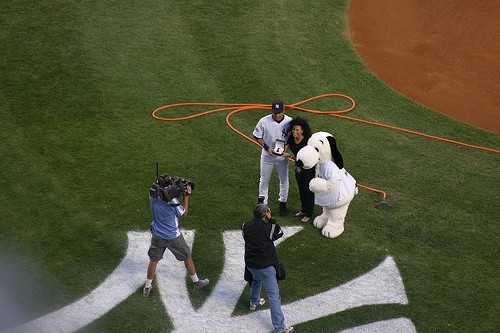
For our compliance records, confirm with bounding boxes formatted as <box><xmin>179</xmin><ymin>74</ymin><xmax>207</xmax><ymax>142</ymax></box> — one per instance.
<box><xmin>296</xmin><ymin>132</ymin><xmax>358</xmax><ymax>238</ymax></box>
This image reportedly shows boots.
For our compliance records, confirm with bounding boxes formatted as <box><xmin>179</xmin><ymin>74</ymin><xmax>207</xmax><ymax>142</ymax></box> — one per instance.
<box><xmin>279</xmin><ymin>202</ymin><xmax>286</xmax><ymax>216</ymax></box>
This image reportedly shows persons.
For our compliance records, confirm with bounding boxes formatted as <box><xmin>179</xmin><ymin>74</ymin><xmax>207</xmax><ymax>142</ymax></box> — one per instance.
<box><xmin>252</xmin><ymin>100</ymin><xmax>293</xmax><ymax>218</ymax></box>
<box><xmin>241</xmin><ymin>205</ymin><xmax>293</xmax><ymax>333</ymax></box>
<box><xmin>143</xmin><ymin>175</ymin><xmax>210</xmax><ymax>296</ymax></box>
<box><xmin>282</xmin><ymin>117</ymin><xmax>315</xmax><ymax>222</ymax></box>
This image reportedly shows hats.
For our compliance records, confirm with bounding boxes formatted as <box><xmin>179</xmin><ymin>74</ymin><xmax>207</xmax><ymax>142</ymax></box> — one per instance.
<box><xmin>271</xmin><ymin>100</ymin><xmax>283</xmax><ymax>113</ymax></box>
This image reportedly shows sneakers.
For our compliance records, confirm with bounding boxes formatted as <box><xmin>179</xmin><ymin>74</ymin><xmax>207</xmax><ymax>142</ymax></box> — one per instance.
<box><xmin>193</xmin><ymin>279</ymin><xmax>210</xmax><ymax>290</ymax></box>
<box><xmin>274</xmin><ymin>326</ymin><xmax>293</xmax><ymax>333</ymax></box>
<box><xmin>143</xmin><ymin>285</ymin><xmax>152</xmax><ymax>298</ymax></box>
<box><xmin>250</xmin><ymin>297</ymin><xmax>266</xmax><ymax>310</ymax></box>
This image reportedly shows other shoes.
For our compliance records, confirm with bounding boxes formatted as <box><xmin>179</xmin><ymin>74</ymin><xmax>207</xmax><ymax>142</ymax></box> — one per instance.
<box><xmin>300</xmin><ymin>216</ymin><xmax>311</xmax><ymax>222</ymax></box>
<box><xmin>295</xmin><ymin>211</ymin><xmax>306</xmax><ymax>216</ymax></box>
